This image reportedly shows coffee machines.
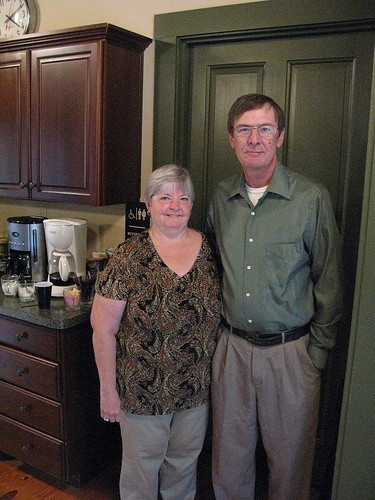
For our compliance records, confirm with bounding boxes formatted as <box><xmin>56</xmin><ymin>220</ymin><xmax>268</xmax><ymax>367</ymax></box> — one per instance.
<box><xmin>4</xmin><ymin>215</ymin><xmax>47</xmax><ymax>283</ymax></box>
<box><xmin>42</xmin><ymin>218</ymin><xmax>87</xmax><ymax>297</ymax></box>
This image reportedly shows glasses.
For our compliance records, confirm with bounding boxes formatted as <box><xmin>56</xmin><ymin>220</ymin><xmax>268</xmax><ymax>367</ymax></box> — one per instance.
<box><xmin>232</xmin><ymin>126</ymin><xmax>279</xmax><ymax>137</ymax></box>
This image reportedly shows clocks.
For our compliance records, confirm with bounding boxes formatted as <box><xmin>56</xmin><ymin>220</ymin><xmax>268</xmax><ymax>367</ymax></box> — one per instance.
<box><xmin>0</xmin><ymin>0</ymin><xmax>37</xmax><ymax>37</ymax></box>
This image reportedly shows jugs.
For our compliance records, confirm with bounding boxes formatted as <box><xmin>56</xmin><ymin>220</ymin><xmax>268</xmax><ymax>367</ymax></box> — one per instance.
<box><xmin>46</xmin><ymin>251</ymin><xmax>76</xmax><ymax>286</ymax></box>
<box><xmin>10</xmin><ymin>253</ymin><xmax>31</xmax><ymax>277</ymax></box>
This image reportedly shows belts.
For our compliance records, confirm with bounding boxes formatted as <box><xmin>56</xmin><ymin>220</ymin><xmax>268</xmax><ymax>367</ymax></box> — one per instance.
<box><xmin>221</xmin><ymin>316</ymin><xmax>310</xmax><ymax>347</ymax></box>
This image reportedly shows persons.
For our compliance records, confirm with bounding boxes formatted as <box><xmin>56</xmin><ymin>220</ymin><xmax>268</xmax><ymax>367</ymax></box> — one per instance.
<box><xmin>187</xmin><ymin>93</ymin><xmax>347</xmax><ymax>500</ymax></box>
<box><xmin>89</xmin><ymin>163</ymin><xmax>223</xmax><ymax>500</ymax></box>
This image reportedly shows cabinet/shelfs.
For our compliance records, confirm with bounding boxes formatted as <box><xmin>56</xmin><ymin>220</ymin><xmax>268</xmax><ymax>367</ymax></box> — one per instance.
<box><xmin>0</xmin><ymin>22</ymin><xmax>153</xmax><ymax>206</ymax></box>
<box><xmin>0</xmin><ymin>315</ymin><xmax>120</xmax><ymax>487</ymax></box>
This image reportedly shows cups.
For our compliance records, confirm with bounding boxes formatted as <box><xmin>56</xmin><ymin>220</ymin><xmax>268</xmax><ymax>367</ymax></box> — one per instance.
<box><xmin>62</xmin><ymin>288</ymin><xmax>81</xmax><ymax>311</ymax></box>
<box><xmin>17</xmin><ymin>283</ymin><xmax>36</xmax><ymax>302</ymax></box>
<box><xmin>34</xmin><ymin>282</ymin><xmax>52</xmax><ymax>309</ymax></box>
<box><xmin>0</xmin><ymin>276</ymin><xmax>19</xmax><ymax>297</ymax></box>
